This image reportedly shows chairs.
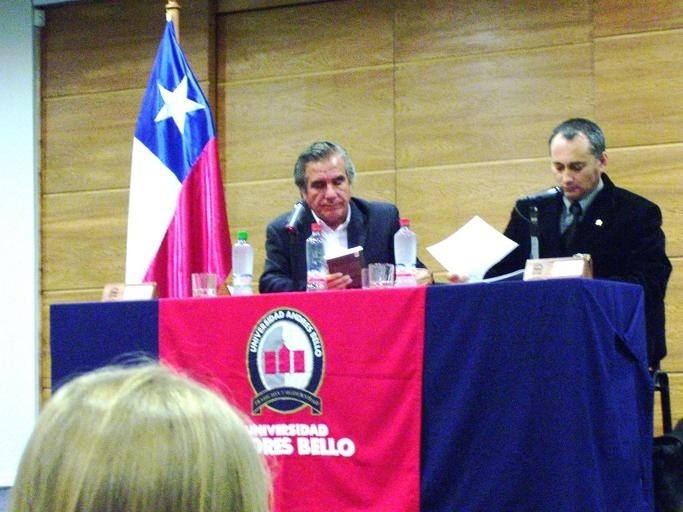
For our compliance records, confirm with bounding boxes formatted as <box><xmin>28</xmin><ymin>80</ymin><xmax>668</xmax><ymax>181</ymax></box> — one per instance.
<box><xmin>654</xmin><ymin>372</ymin><xmax>674</xmax><ymax>439</ymax></box>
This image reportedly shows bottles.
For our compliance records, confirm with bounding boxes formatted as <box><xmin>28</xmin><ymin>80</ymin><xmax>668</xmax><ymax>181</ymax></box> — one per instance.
<box><xmin>394</xmin><ymin>218</ymin><xmax>417</xmax><ymax>288</ymax></box>
<box><xmin>306</xmin><ymin>223</ymin><xmax>327</xmax><ymax>292</ymax></box>
<box><xmin>231</xmin><ymin>231</ymin><xmax>254</xmax><ymax>297</ymax></box>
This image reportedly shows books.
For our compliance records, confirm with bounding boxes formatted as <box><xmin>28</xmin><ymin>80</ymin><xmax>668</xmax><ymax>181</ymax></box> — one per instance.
<box><xmin>323</xmin><ymin>245</ymin><xmax>365</xmax><ymax>289</ymax></box>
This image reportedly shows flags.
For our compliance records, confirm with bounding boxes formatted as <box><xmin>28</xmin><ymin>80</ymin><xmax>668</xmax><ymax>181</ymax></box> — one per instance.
<box><xmin>118</xmin><ymin>18</ymin><xmax>233</xmax><ymax>297</ymax></box>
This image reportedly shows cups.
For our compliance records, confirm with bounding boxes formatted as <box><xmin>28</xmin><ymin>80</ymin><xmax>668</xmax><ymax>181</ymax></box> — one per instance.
<box><xmin>361</xmin><ymin>264</ymin><xmax>394</xmax><ymax>289</ymax></box>
<box><xmin>191</xmin><ymin>273</ymin><xmax>217</xmax><ymax>298</ymax></box>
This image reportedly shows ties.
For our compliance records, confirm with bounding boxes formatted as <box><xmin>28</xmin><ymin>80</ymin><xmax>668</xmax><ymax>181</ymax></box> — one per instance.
<box><xmin>562</xmin><ymin>205</ymin><xmax>581</xmax><ymax>252</ymax></box>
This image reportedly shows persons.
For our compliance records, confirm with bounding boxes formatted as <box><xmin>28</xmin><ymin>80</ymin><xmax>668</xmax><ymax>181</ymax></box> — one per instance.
<box><xmin>256</xmin><ymin>140</ymin><xmax>436</xmax><ymax>291</ymax></box>
<box><xmin>5</xmin><ymin>348</ymin><xmax>276</xmax><ymax>512</ymax></box>
<box><xmin>443</xmin><ymin>116</ymin><xmax>674</xmax><ymax>379</ymax></box>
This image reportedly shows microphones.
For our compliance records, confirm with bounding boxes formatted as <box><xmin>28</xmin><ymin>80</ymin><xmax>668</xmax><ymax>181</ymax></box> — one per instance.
<box><xmin>285</xmin><ymin>201</ymin><xmax>307</xmax><ymax>235</ymax></box>
